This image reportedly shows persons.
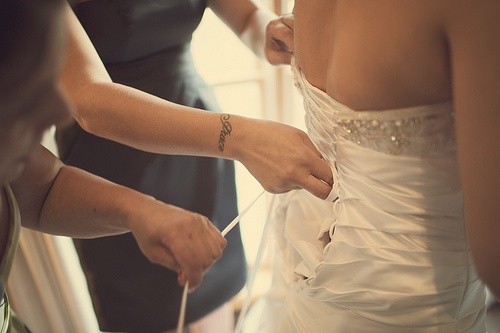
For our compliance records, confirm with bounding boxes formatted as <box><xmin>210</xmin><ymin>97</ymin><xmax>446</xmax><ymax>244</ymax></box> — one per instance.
<box><xmin>53</xmin><ymin>0</ymin><xmax>294</xmax><ymax>332</ymax></box>
<box><xmin>0</xmin><ymin>0</ymin><xmax>230</xmax><ymax>331</ymax></box>
<box><xmin>234</xmin><ymin>1</ymin><xmax>499</xmax><ymax>333</ymax></box>
